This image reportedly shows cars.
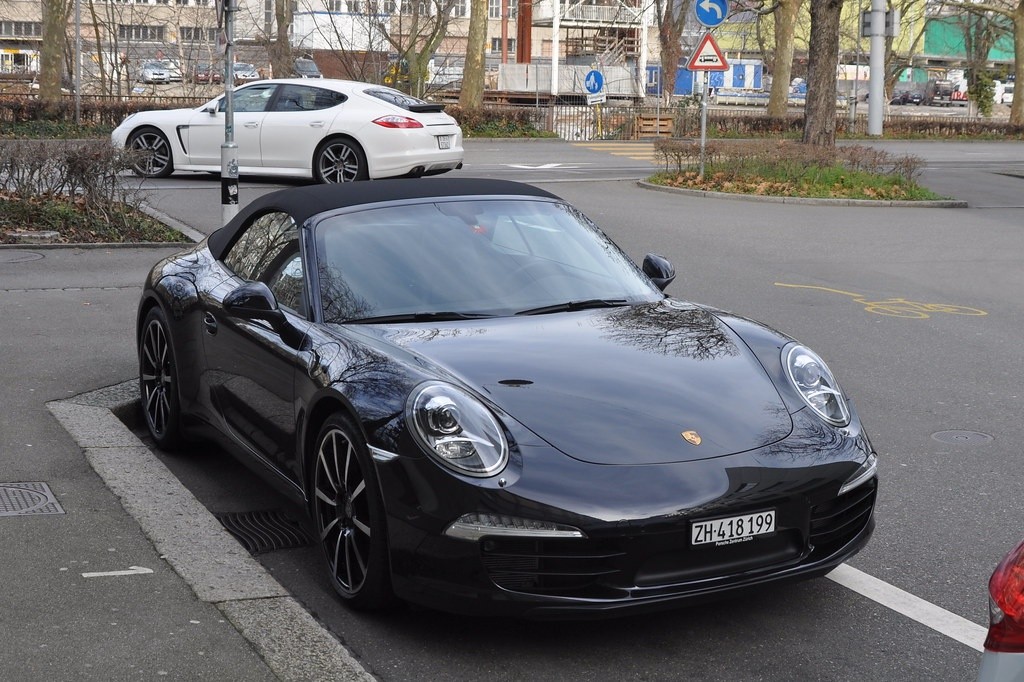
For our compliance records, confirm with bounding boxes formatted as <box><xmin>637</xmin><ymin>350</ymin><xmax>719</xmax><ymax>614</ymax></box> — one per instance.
<box><xmin>111</xmin><ymin>75</ymin><xmax>465</xmax><ymax>183</ymax></box>
<box><xmin>191</xmin><ymin>63</ymin><xmax>222</xmax><ymax>84</ymax></box>
<box><xmin>135</xmin><ymin>58</ymin><xmax>170</xmax><ymax>84</ymax></box>
<box><xmin>886</xmin><ymin>78</ymin><xmax>1017</xmax><ymax>106</ymax></box>
<box><xmin>290</xmin><ymin>59</ymin><xmax>323</xmax><ymax>79</ymax></box>
<box><xmin>222</xmin><ymin>63</ymin><xmax>260</xmax><ymax>87</ymax></box>
<box><xmin>161</xmin><ymin>59</ymin><xmax>188</xmax><ymax>82</ymax></box>
<box><xmin>89</xmin><ymin>52</ymin><xmax>129</xmax><ymax>82</ymax></box>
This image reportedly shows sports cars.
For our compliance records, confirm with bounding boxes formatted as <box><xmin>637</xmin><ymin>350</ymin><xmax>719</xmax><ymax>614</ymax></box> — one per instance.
<box><xmin>135</xmin><ymin>178</ymin><xmax>879</xmax><ymax>625</ymax></box>
